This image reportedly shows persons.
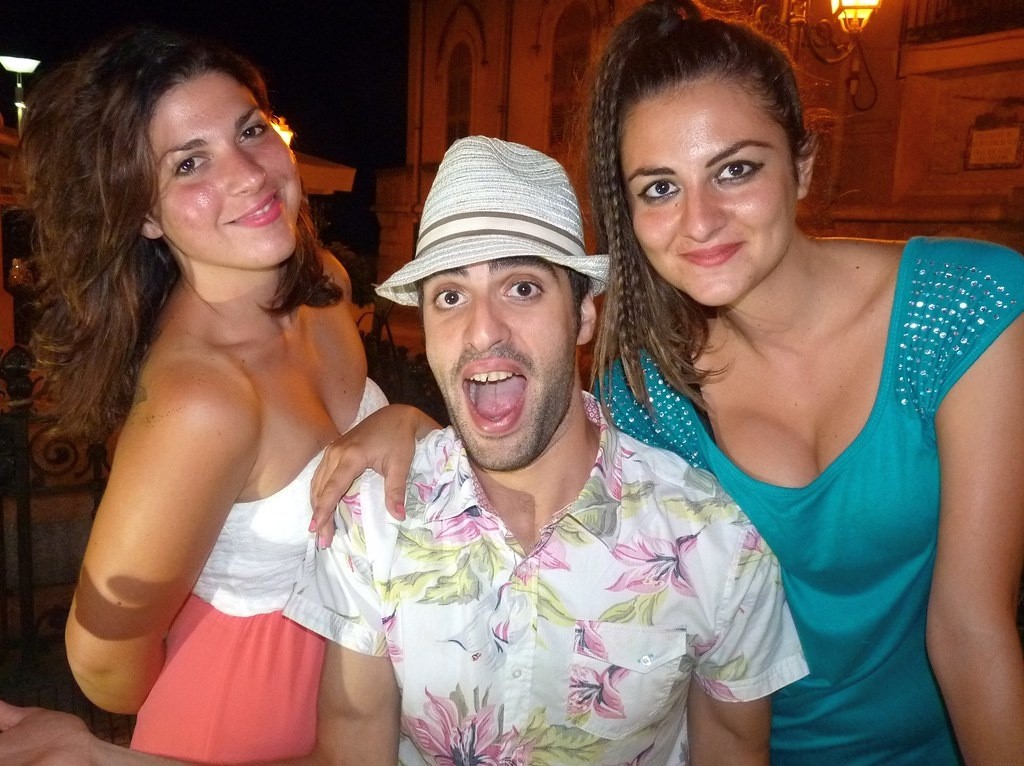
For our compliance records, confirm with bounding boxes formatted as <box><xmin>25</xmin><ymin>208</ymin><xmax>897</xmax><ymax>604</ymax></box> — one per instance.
<box><xmin>17</xmin><ymin>41</ymin><xmax>390</xmax><ymax>766</ymax></box>
<box><xmin>588</xmin><ymin>0</ymin><xmax>1024</xmax><ymax>766</ymax></box>
<box><xmin>0</xmin><ymin>135</ymin><xmax>810</xmax><ymax>765</ymax></box>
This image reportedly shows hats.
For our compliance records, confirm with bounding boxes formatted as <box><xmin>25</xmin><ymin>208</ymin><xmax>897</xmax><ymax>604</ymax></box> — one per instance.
<box><xmin>373</xmin><ymin>135</ymin><xmax>610</xmax><ymax>306</ymax></box>
